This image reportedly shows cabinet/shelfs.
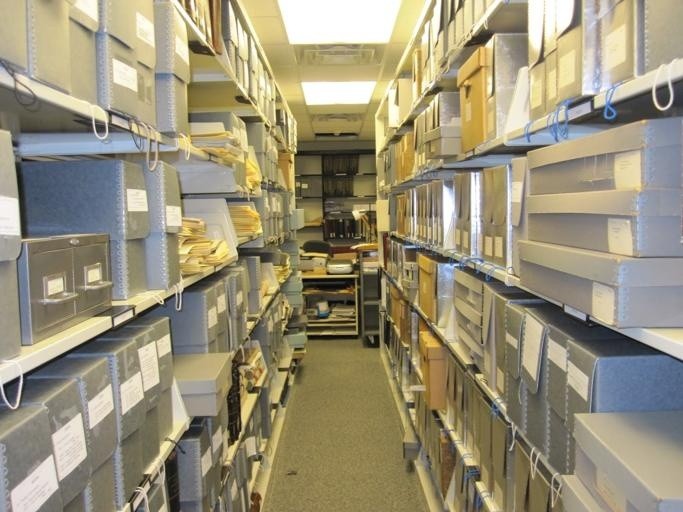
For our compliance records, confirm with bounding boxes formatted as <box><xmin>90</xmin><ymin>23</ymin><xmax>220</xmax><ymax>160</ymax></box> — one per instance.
<box><xmin>292</xmin><ymin>149</ymin><xmax>378</xmax><ymax>345</ymax></box>
<box><xmin>0</xmin><ymin>0</ymin><xmax>306</xmax><ymax>511</ymax></box>
<box><xmin>375</xmin><ymin>1</ymin><xmax>681</xmax><ymax>512</ymax></box>
